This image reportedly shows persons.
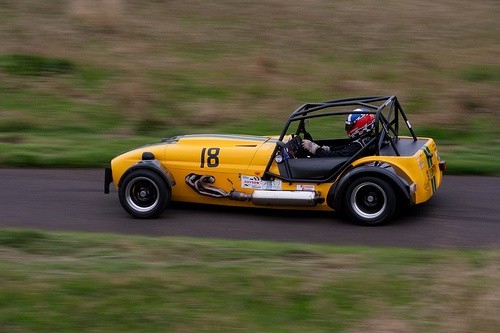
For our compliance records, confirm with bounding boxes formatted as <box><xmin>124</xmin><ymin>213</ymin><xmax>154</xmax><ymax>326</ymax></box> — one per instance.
<box><xmin>300</xmin><ymin>107</ymin><xmax>378</xmax><ymax>159</ymax></box>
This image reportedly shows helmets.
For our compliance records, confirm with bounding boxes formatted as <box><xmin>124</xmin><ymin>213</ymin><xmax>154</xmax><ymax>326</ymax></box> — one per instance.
<box><xmin>345</xmin><ymin>108</ymin><xmax>376</xmax><ymax>138</ymax></box>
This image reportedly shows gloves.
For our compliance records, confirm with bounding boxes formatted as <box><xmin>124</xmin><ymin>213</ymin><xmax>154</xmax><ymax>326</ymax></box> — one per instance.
<box><xmin>301</xmin><ymin>139</ymin><xmax>320</xmax><ymax>154</ymax></box>
<box><xmin>322</xmin><ymin>145</ymin><xmax>330</xmax><ymax>151</ymax></box>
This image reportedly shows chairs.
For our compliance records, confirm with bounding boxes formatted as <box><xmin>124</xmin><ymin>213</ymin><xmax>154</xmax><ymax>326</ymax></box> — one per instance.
<box><xmin>359</xmin><ymin>145</ymin><xmax>376</xmax><ymax>156</ymax></box>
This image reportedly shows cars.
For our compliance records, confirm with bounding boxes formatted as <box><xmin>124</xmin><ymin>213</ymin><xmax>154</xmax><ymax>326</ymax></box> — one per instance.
<box><xmin>104</xmin><ymin>95</ymin><xmax>448</xmax><ymax>228</ymax></box>
